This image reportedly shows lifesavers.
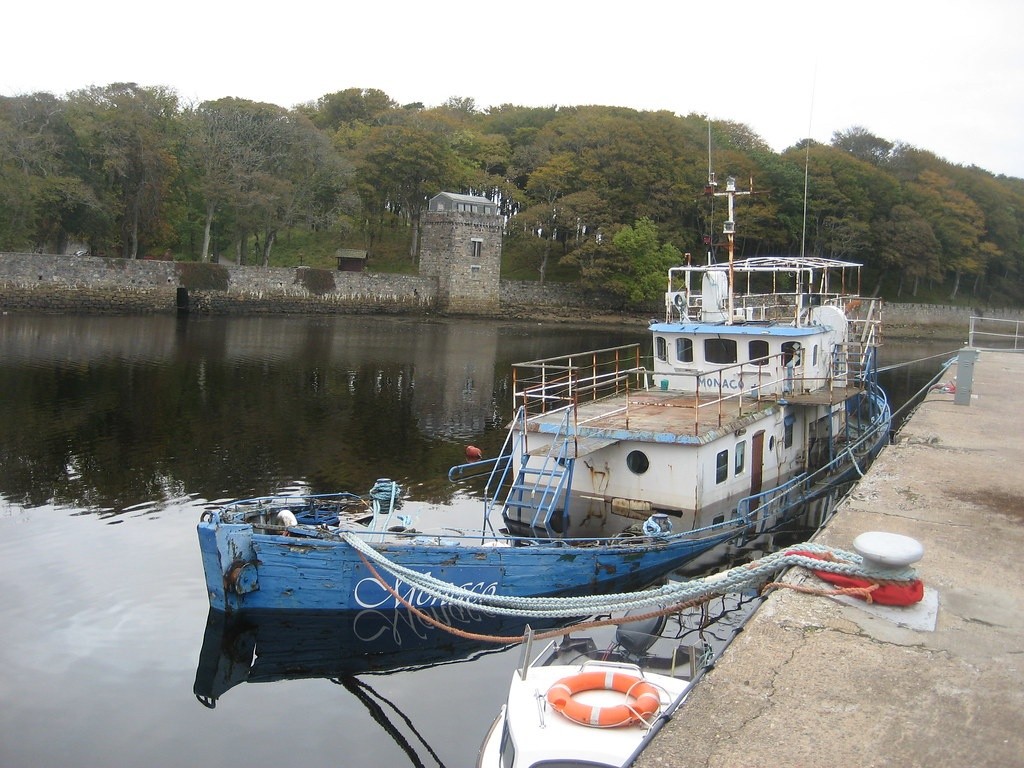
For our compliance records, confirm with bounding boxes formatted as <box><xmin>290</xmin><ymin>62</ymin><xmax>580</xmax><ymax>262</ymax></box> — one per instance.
<box><xmin>546</xmin><ymin>671</ymin><xmax>661</xmax><ymax>727</ymax></box>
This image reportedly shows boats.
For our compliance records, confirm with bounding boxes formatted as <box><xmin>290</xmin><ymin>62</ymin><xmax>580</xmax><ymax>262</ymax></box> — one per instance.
<box><xmin>476</xmin><ymin>624</ymin><xmax>715</xmax><ymax>767</ymax></box>
<box><xmin>193</xmin><ymin>486</ymin><xmax>845</xmax><ymax>712</ymax></box>
<box><xmin>197</xmin><ymin>164</ymin><xmax>894</xmax><ymax>618</ymax></box>
